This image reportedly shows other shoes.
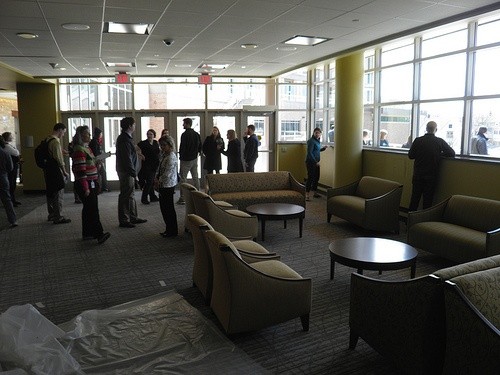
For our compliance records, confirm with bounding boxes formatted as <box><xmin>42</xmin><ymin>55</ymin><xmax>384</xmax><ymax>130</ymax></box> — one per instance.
<box><xmin>130</xmin><ymin>218</ymin><xmax>147</xmax><ymax>223</ymax></box>
<box><xmin>150</xmin><ymin>195</ymin><xmax>159</xmax><ymax>201</ymax></box>
<box><xmin>82</xmin><ymin>234</ymin><xmax>96</xmax><ymax>240</ymax></box>
<box><xmin>15</xmin><ymin>201</ymin><xmax>21</xmax><ymax>205</ymax></box>
<box><xmin>76</xmin><ymin>200</ymin><xmax>82</xmax><ymax>203</ymax></box>
<box><xmin>305</xmin><ymin>195</ymin><xmax>309</xmax><ymax>200</ymax></box>
<box><xmin>12</xmin><ymin>222</ymin><xmax>19</xmax><ymax>226</ymax></box>
<box><xmin>99</xmin><ymin>232</ymin><xmax>111</xmax><ymax>242</ymax></box>
<box><xmin>53</xmin><ymin>219</ymin><xmax>71</xmax><ymax>224</ymax></box>
<box><xmin>313</xmin><ymin>193</ymin><xmax>322</xmax><ymax>198</ymax></box>
<box><xmin>160</xmin><ymin>230</ymin><xmax>177</xmax><ymax>237</ymax></box>
<box><xmin>48</xmin><ymin>216</ymin><xmax>64</xmax><ymax>220</ymax></box>
<box><xmin>119</xmin><ymin>222</ymin><xmax>136</xmax><ymax>228</ymax></box>
<box><xmin>141</xmin><ymin>199</ymin><xmax>150</xmax><ymax>204</ymax></box>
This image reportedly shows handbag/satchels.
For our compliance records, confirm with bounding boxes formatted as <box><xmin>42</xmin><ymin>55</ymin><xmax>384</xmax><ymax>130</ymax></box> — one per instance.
<box><xmin>154</xmin><ymin>174</ymin><xmax>159</xmax><ymax>192</ymax></box>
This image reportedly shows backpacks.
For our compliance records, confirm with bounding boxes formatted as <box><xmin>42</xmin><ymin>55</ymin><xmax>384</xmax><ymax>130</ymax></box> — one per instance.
<box><xmin>35</xmin><ymin>138</ymin><xmax>56</xmax><ymax>170</ymax></box>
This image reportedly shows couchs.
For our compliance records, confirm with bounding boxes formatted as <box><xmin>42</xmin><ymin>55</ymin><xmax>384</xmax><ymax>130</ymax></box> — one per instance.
<box><xmin>180</xmin><ymin>182</ymin><xmax>233</xmax><ymax>233</ymax></box>
<box><xmin>407</xmin><ymin>194</ymin><xmax>500</xmax><ymax>265</ymax></box>
<box><xmin>349</xmin><ymin>255</ymin><xmax>500</xmax><ymax>375</ymax></box>
<box><xmin>191</xmin><ymin>190</ymin><xmax>259</xmax><ymax>242</ymax></box>
<box><xmin>327</xmin><ymin>176</ymin><xmax>404</xmax><ymax>237</ymax></box>
<box><xmin>441</xmin><ymin>267</ymin><xmax>500</xmax><ymax>375</ymax></box>
<box><xmin>186</xmin><ymin>213</ymin><xmax>277</xmax><ymax>306</ymax></box>
<box><xmin>203</xmin><ymin>171</ymin><xmax>306</xmax><ymax>219</ymax></box>
<box><xmin>204</xmin><ymin>230</ymin><xmax>312</xmax><ymax>341</ymax></box>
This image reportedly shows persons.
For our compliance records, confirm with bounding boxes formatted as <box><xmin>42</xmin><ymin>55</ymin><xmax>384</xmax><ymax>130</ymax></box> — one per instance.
<box><xmin>402</xmin><ymin>136</ymin><xmax>413</xmax><ymax>148</ymax></box>
<box><xmin>117</xmin><ymin>117</ymin><xmax>147</xmax><ymax>228</ymax></box>
<box><xmin>154</xmin><ymin>136</ymin><xmax>177</xmax><ymax>237</ymax></box>
<box><xmin>42</xmin><ymin>123</ymin><xmax>71</xmax><ymax>224</ymax></box>
<box><xmin>178</xmin><ymin>118</ymin><xmax>202</xmax><ymax>204</ymax></box>
<box><xmin>138</xmin><ymin>128</ymin><xmax>176</xmax><ymax>204</ymax></box>
<box><xmin>363</xmin><ymin>129</ymin><xmax>372</xmax><ymax>145</ymax></box>
<box><xmin>221</xmin><ymin>129</ymin><xmax>243</xmax><ymax>173</ymax></box>
<box><xmin>471</xmin><ymin>127</ymin><xmax>489</xmax><ymax>154</ymax></box>
<box><xmin>305</xmin><ymin>128</ymin><xmax>327</xmax><ymax>200</ymax></box>
<box><xmin>0</xmin><ymin>132</ymin><xmax>23</xmax><ymax>226</ymax></box>
<box><xmin>328</xmin><ymin>125</ymin><xmax>334</xmax><ymax>142</ymax></box>
<box><xmin>404</xmin><ymin>121</ymin><xmax>455</xmax><ymax>229</ymax></box>
<box><xmin>204</xmin><ymin>127</ymin><xmax>225</xmax><ymax>174</ymax></box>
<box><xmin>243</xmin><ymin>125</ymin><xmax>259</xmax><ymax>172</ymax></box>
<box><xmin>380</xmin><ymin>130</ymin><xmax>389</xmax><ymax>146</ymax></box>
<box><xmin>68</xmin><ymin>126</ymin><xmax>112</xmax><ymax>243</ymax></box>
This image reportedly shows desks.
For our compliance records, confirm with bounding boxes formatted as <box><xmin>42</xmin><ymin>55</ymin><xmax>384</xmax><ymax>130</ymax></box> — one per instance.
<box><xmin>328</xmin><ymin>237</ymin><xmax>419</xmax><ymax>281</ymax></box>
<box><xmin>246</xmin><ymin>203</ymin><xmax>305</xmax><ymax>243</ymax></box>
